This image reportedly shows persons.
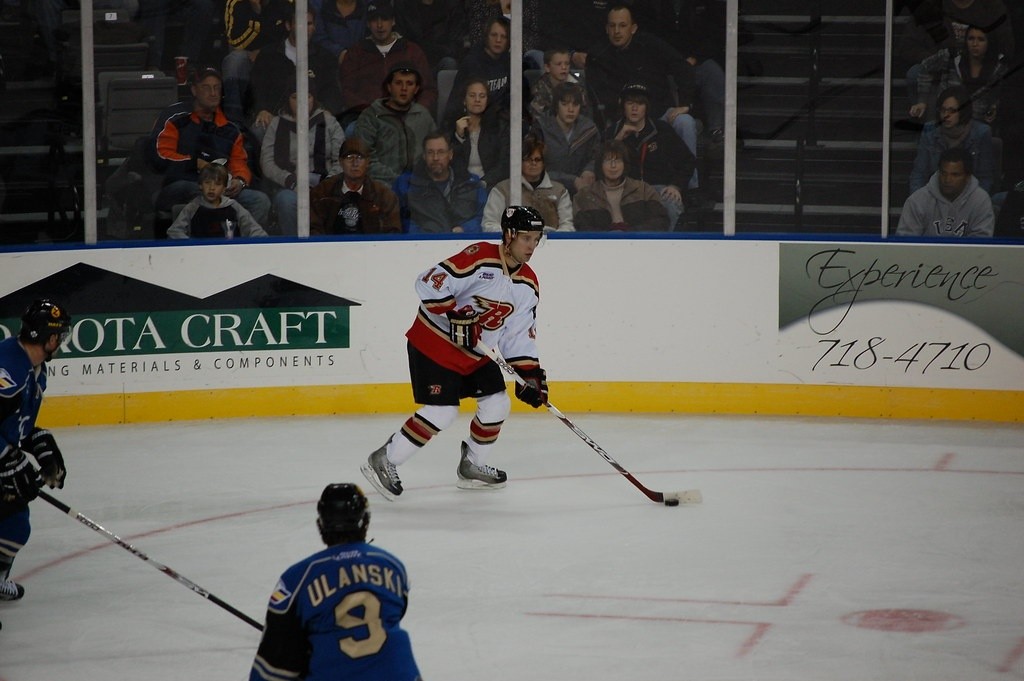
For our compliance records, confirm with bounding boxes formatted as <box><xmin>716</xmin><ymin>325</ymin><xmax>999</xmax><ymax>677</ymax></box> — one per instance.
<box><xmin>249</xmin><ymin>483</ymin><xmax>423</xmax><ymax>681</ymax></box>
<box><xmin>0</xmin><ymin>300</ymin><xmax>67</xmax><ymax>630</ymax></box>
<box><xmin>133</xmin><ymin>0</ymin><xmax>741</xmax><ymax>240</ymax></box>
<box><xmin>895</xmin><ymin>0</ymin><xmax>1024</xmax><ymax>238</ymax></box>
<box><xmin>368</xmin><ymin>205</ymin><xmax>549</xmax><ymax>496</ymax></box>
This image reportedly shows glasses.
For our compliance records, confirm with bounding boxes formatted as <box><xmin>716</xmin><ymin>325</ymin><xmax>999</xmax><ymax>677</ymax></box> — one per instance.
<box><xmin>345</xmin><ymin>153</ymin><xmax>364</xmax><ymax>159</ymax></box>
<box><xmin>938</xmin><ymin>106</ymin><xmax>960</xmax><ymax>114</ymax></box>
<box><xmin>522</xmin><ymin>156</ymin><xmax>544</xmax><ymax>162</ymax></box>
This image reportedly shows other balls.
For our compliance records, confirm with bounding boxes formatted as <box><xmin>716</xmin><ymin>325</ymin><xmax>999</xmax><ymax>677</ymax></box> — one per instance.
<box><xmin>665</xmin><ymin>499</ymin><xmax>679</xmax><ymax>506</ymax></box>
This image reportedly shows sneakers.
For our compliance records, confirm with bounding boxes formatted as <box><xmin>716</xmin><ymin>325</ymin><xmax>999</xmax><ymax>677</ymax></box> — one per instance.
<box><xmin>455</xmin><ymin>440</ymin><xmax>508</xmax><ymax>490</ymax></box>
<box><xmin>0</xmin><ymin>553</ymin><xmax>25</xmax><ymax>598</ymax></box>
<box><xmin>360</xmin><ymin>432</ymin><xmax>405</xmax><ymax>502</ymax></box>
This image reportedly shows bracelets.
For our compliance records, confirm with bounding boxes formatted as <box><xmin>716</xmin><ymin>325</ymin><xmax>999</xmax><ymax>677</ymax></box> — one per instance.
<box><xmin>235</xmin><ymin>177</ymin><xmax>246</xmax><ymax>192</ymax></box>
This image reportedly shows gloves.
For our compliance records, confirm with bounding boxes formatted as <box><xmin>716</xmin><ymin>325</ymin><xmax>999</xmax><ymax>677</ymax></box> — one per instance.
<box><xmin>0</xmin><ymin>443</ymin><xmax>41</xmax><ymax>506</ymax></box>
<box><xmin>447</xmin><ymin>305</ymin><xmax>482</xmax><ymax>347</ymax></box>
<box><xmin>512</xmin><ymin>364</ymin><xmax>549</xmax><ymax>407</ymax></box>
<box><xmin>24</xmin><ymin>428</ymin><xmax>66</xmax><ymax>490</ymax></box>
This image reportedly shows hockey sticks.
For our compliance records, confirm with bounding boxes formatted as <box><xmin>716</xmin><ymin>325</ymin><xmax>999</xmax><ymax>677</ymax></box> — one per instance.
<box><xmin>476</xmin><ymin>338</ymin><xmax>703</xmax><ymax>505</ymax></box>
<box><xmin>37</xmin><ymin>488</ymin><xmax>264</xmax><ymax>632</ymax></box>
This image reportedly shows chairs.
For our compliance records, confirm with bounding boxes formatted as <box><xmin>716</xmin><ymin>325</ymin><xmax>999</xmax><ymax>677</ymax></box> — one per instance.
<box><xmin>0</xmin><ymin>0</ymin><xmax>1024</xmax><ymax>242</ymax></box>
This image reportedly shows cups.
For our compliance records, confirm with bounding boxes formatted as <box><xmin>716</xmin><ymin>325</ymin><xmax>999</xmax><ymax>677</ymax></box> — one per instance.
<box><xmin>175</xmin><ymin>56</ymin><xmax>189</xmax><ymax>85</ymax></box>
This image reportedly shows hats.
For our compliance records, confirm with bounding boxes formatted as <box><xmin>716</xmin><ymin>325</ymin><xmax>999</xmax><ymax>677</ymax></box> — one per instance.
<box><xmin>195</xmin><ymin>62</ymin><xmax>221</xmax><ymax>81</ymax></box>
<box><xmin>277</xmin><ymin>67</ymin><xmax>316</xmax><ymax>113</ymax></box>
<box><xmin>624</xmin><ymin>83</ymin><xmax>647</xmax><ymax>104</ymax></box>
<box><xmin>382</xmin><ymin>60</ymin><xmax>423</xmax><ymax>93</ymax></box>
<box><xmin>340</xmin><ymin>137</ymin><xmax>369</xmax><ymax>156</ymax></box>
<box><xmin>366</xmin><ymin>0</ymin><xmax>394</xmax><ymax>17</ymax></box>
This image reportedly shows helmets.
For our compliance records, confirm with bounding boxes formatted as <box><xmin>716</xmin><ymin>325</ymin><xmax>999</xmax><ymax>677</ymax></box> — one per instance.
<box><xmin>500</xmin><ymin>206</ymin><xmax>547</xmax><ymax>231</ymax></box>
<box><xmin>21</xmin><ymin>295</ymin><xmax>70</xmax><ymax>331</ymax></box>
<box><xmin>317</xmin><ymin>484</ymin><xmax>370</xmax><ymax>531</ymax></box>
